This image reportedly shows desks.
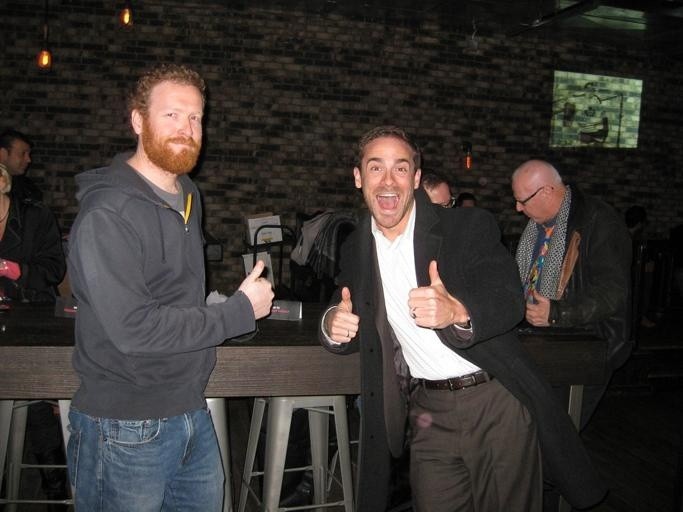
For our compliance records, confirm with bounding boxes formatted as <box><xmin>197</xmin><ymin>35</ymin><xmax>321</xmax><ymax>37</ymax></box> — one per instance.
<box><xmin>0</xmin><ymin>299</ymin><xmax>592</xmax><ymax>510</ymax></box>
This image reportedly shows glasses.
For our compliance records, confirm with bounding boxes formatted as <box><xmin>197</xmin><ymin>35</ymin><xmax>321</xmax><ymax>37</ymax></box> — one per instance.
<box><xmin>440</xmin><ymin>195</ymin><xmax>455</xmax><ymax>209</ymax></box>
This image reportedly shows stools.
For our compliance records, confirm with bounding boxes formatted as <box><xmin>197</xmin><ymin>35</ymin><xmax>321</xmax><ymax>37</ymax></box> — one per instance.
<box><xmin>327</xmin><ymin>392</ymin><xmax>364</xmax><ymax>503</ymax></box>
<box><xmin>200</xmin><ymin>397</ymin><xmax>233</xmax><ymax>510</ymax></box>
<box><xmin>236</xmin><ymin>395</ymin><xmax>356</xmax><ymax>510</ymax></box>
<box><xmin>1</xmin><ymin>399</ymin><xmax>79</xmax><ymax>509</ymax></box>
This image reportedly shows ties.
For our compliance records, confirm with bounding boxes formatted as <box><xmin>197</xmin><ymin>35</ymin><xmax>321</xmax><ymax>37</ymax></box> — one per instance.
<box><xmin>524</xmin><ymin>225</ymin><xmax>555</xmax><ymax>303</ymax></box>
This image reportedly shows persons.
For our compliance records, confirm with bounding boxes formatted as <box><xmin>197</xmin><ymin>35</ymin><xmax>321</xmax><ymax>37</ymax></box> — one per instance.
<box><xmin>0</xmin><ymin>162</ymin><xmax>71</xmax><ymax>512</ymax></box>
<box><xmin>313</xmin><ymin>121</ymin><xmax>609</xmax><ymax>510</ymax></box>
<box><xmin>420</xmin><ymin>172</ymin><xmax>458</xmax><ymax>210</ymax></box>
<box><xmin>63</xmin><ymin>60</ymin><xmax>279</xmax><ymax>512</ymax></box>
<box><xmin>0</xmin><ymin>128</ymin><xmax>45</xmax><ymax>198</ymax></box>
<box><xmin>455</xmin><ymin>191</ymin><xmax>479</xmax><ymax>208</ymax></box>
<box><xmin>509</xmin><ymin>158</ymin><xmax>634</xmax><ymax>431</ymax></box>
<box><xmin>623</xmin><ymin>206</ymin><xmax>658</xmax><ymax>330</ymax></box>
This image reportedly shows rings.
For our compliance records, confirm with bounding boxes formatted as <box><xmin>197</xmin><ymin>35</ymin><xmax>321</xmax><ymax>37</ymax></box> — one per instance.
<box><xmin>410</xmin><ymin>306</ymin><xmax>420</xmax><ymax>319</ymax></box>
<box><xmin>344</xmin><ymin>329</ymin><xmax>352</xmax><ymax>338</ymax></box>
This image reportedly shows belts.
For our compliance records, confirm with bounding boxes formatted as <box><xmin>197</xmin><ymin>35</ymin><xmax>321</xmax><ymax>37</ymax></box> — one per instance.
<box><xmin>417</xmin><ymin>370</ymin><xmax>492</xmax><ymax>391</ymax></box>
